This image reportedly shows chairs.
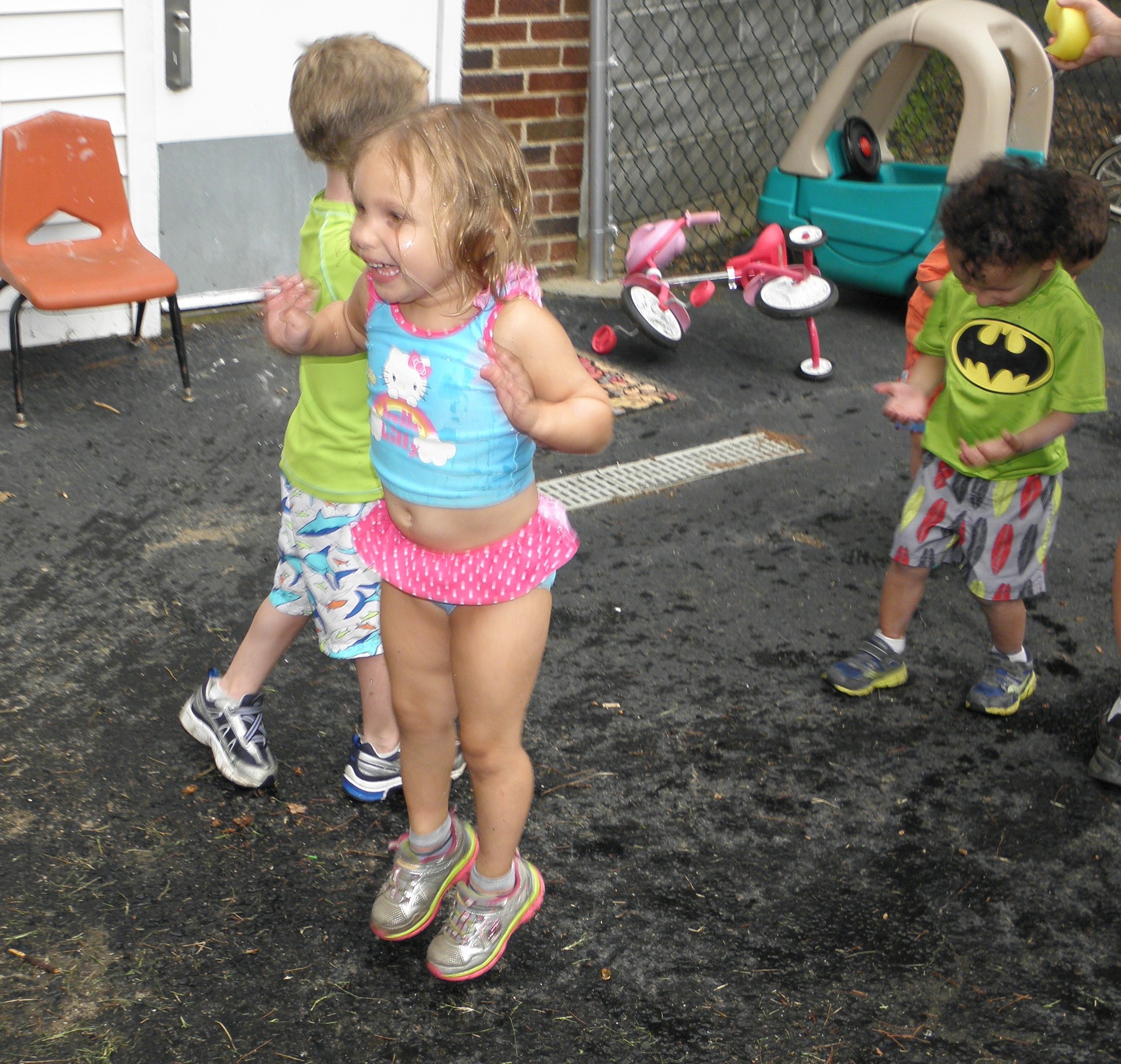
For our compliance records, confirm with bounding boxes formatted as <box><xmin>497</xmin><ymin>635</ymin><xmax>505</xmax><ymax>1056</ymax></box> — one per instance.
<box><xmin>0</xmin><ymin>111</ymin><xmax>195</xmax><ymax>428</ymax></box>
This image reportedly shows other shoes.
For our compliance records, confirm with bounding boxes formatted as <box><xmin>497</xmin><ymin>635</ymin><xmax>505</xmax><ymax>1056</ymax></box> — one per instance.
<box><xmin>1087</xmin><ymin>706</ymin><xmax>1120</xmax><ymax>788</ymax></box>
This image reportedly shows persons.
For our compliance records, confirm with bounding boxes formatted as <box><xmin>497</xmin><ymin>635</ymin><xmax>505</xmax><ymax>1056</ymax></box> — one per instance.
<box><xmin>263</xmin><ymin>100</ymin><xmax>616</xmax><ymax>981</ymax></box>
<box><xmin>823</xmin><ymin>0</ymin><xmax>1121</xmax><ymax>787</ymax></box>
<box><xmin>180</xmin><ymin>34</ymin><xmax>466</xmax><ymax>803</ymax></box>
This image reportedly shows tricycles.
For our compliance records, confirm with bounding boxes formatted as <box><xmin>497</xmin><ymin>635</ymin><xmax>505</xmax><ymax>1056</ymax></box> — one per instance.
<box><xmin>588</xmin><ymin>209</ymin><xmax>837</xmax><ymax>381</ymax></box>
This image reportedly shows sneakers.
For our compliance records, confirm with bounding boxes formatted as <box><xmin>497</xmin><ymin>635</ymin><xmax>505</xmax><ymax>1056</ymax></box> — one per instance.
<box><xmin>342</xmin><ymin>731</ymin><xmax>466</xmax><ymax>803</ymax></box>
<box><xmin>368</xmin><ymin>805</ymin><xmax>484</xmax><ymax>944</ymax></box>
<box><xmin>180</xmin><ymin>667</ymin><xmax>279</xmax><ymax>789</ymax></box>
<box><xmin>966</xmin><ymin>647</ymin><xmax>1037</xmax><ymax>717</ymax></box>
<box><xmin>827</xmin><ymin>633</ymin><xmax>907</xmax><ymax>696</ymax></box>
<box><xmin>428</xmin><ymin>847</ymin><xmax>545</xmax><ymax>982</ymax></box>
